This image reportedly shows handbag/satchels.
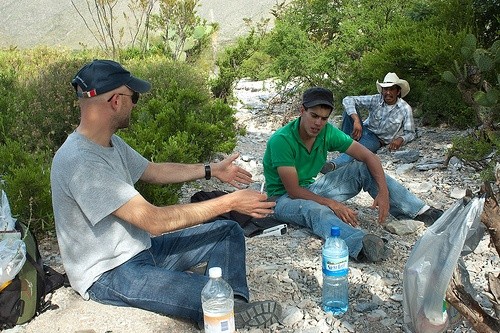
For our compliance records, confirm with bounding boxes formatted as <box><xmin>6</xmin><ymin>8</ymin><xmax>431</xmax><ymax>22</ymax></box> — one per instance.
<box><xmin>0</xmin><ymin>188</ymin><xmax>65</xmax><ymax>330</ymax></box>
<box><xmin>402</xmin><ymin>198</ymin><xmax>485</xmax><ymax>333</ymax></box>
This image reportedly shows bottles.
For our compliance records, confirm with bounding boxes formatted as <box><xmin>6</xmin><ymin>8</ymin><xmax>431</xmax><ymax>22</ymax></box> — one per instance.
<box><xmin>321</xmin><ymin>226</ymin><xmax>349</xmax><ymax>316</ymax></box>
<box><xmin>201</xmin><ymin>267</ymin><xmax>235</xmax><ymax>333</ymax></box>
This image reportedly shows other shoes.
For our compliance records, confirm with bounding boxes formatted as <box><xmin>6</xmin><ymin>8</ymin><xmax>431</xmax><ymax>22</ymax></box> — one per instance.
<box><xmin>320</xmin><ymin>162</ymin><xmax>333</xmax><ymax>174</ymax></box>
<box><xmin>234</xmin><ymin>298</ymin><xmax>282</xmax><ymax>330</ymax></box>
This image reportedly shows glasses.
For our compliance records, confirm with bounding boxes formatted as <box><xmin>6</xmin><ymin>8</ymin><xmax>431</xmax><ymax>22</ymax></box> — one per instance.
<box><xmin>107</xmin><ymin>89</ymin><xmax>140</xmax><ymax>104</ymax></box>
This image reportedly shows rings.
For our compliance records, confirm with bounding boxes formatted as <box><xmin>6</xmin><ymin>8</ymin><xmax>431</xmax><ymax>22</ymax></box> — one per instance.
<box><xmin>395</xmin><ymin>148</ymin><xmax>396</xmax><ymax>149</ymax></box>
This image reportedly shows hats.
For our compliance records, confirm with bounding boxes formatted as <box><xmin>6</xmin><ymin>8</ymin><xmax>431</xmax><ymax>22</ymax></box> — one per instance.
<box><xmin>376</xmin><ymin>72</ymin><xmax>410</xmax><ymax>98</ymax></box>
<box><xmin>303</xmin><ymin>87</ymin><xmax>334</xmax><ymax>109</ymax></box>
<box><xmin>71</xmin><ymin>59</ymin><xmax>152</xmax><ymax>97</ymax></box>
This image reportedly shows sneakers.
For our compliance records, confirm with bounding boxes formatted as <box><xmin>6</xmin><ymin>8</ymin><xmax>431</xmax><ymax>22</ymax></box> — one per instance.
<box><xmin>414</xmin><ymin>206</ymin><xmax>445</xmax><ymax>225</ymax></box>
<box><xmin>362</xmin><ymin>234</ymin><xmax>385</xmax><ymax>262</ymax></box>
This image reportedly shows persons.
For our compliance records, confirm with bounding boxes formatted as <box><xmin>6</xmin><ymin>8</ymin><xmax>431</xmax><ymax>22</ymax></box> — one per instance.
<box><xmin>319</xmin><ymin>72</ymin><xmax>416</xmax><ymax>174</ymax></box>
<box><xmin>50</xmin><ymin>59</ymin><xmax>282</xmax><ymax>333</ymax></box>
<box><xmin>262</xmin><ymin>87</ymin><xmax>444</xmax><ymax>262</ymax></box>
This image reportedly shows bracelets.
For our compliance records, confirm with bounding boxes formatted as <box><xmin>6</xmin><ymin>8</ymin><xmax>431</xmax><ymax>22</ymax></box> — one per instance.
<box><xmin>204</xmin><ymin>164</ymin><xmax>211</xmax><ymax>180</ymax></box>
